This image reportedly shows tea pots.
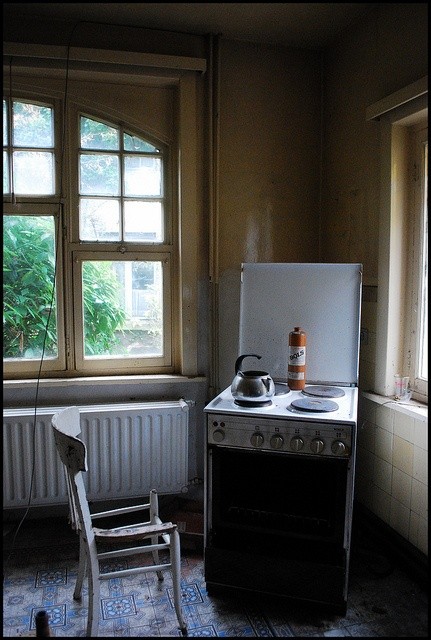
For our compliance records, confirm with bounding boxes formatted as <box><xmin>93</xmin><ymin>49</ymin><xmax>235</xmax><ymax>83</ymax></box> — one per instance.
<box><xmin>231</xmin><ymin>353</ymin><xmax>276</xmax><ymax>406</ymax></box>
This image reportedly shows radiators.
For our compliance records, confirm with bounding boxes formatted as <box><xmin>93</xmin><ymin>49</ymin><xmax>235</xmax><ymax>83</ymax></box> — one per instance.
<box><xmin>2</xmin><ymin>397</ymin><xmax>197</xmax><ymax>510</ymax></box>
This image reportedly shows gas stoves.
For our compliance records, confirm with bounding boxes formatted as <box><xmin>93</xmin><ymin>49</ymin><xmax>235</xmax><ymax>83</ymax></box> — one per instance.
<box><xmin>201</xmin><ymin>262</ymin><xmax>366</xmax><ymax>449</ymax></box>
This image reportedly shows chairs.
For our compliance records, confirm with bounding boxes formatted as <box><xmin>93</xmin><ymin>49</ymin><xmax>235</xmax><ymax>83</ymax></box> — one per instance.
<box><xmin>51</xmin><ymin>406</ymin><xmax>187</xmax><ymax>637</ymax></box>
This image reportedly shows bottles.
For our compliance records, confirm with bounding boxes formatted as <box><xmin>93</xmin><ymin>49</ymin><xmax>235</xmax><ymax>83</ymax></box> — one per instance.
<box><xmin>287</xmin><ymin>325</ymin><xmax>308</xmax><ymax>392</ymax></box>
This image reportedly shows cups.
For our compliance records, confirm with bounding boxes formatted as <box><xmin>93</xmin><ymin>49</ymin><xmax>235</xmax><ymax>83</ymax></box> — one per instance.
<box><xmin>393</xmin><ymin>376</ymin><xmax>411</xmax><ymax>402</ymax></box>
